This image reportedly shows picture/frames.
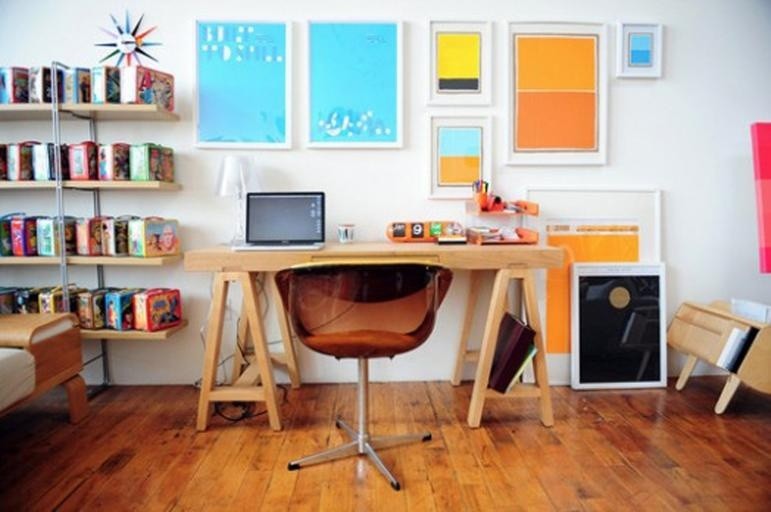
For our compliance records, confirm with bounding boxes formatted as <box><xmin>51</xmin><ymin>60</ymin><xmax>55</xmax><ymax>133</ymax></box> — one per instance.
<box><xmin>193</xmin><ymin>18</ymin><xmax>290</xmax><ymax>148</ymax></box>
<box><xmin>426</xmin><ymin>116</ymin><xmax>495</xmax><ymax>202</ymax></box>
<box><xmin>568</xmin><ymin>262</ymin><xmax>667</xmax><ymax>391</ymax></box>
<box><xmin>611</xmin><ymin>21</ymin><xmax>663</xmax><ymax>80</ymax></box>
<box><xmin>520</xmin><ymin>186</ymin><xmax>663</xmax><ymax>385</ymax></box>
<box><xmin>304</xmin><ymin>18</ymin><xmax>401</xmax><ymax>147</ymax></box>
<box><xmin>426</xmin><ymin>20</ymin><xmax>495</xmax><ymax>108</ymax></box>
<box><xmin>507</xmin><ymin>20</ymin><xmax>611</xmax><ymax>167</ymax></box>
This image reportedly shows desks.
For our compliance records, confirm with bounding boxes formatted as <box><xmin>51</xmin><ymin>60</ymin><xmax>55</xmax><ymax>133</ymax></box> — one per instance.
<box><xmin>183</xmin><ymin>239</ymin><xmax>568</xmax><ymax>434</ymax></box>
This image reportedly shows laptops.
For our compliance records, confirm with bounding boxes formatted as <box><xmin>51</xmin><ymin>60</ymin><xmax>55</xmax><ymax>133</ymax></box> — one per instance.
<box><xmin>231</xmin><ymin>191</ymin><xmax>327</xmax><ymax>251</ymax></box>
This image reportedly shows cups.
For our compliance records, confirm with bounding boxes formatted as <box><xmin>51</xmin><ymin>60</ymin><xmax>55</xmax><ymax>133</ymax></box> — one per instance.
<box><xmin>336</xmin><ymin>223</ymin><xmax>357</xmax><ymax>244</ymax></box>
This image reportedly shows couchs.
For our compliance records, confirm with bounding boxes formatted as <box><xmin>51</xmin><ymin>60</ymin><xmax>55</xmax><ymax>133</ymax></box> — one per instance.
<box><xmin>0</xmin><ymin>310</ymin><xmax>88</xmax><ymax>425</ymax></box>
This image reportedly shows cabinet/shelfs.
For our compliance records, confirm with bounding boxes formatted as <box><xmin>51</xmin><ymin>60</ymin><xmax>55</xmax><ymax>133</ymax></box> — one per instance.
<box><xmin>0</xmin><ymin>62</ymin><xmax>182</xmax><ymax>402</ymax></box>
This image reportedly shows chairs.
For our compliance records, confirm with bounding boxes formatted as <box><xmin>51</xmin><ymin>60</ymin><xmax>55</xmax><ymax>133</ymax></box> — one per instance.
<box><xmin>273</xmin><ymin>261</ymin><xmax>452</xmax><ymax>493</ymax></box>
